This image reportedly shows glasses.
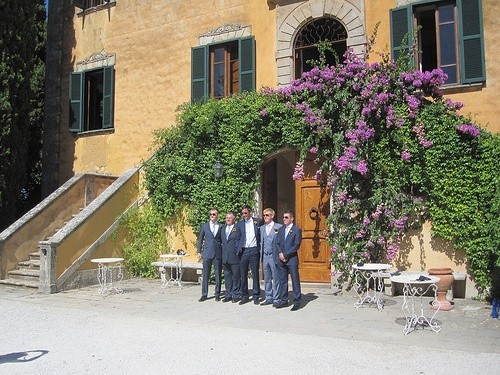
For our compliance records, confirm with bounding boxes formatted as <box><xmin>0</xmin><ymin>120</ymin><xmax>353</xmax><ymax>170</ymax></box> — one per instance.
<box><xmin>242</xmin><ymin>213</ymin><xmax>248</xmax><ymax>215</ymax></box>
<box><xmin>210</xmin><ymin>214</ymin><xmax>216</xmax><ymax>216</ymax></box>
<box><xmin>263</xmin><ymin>214</ymin><xmax>272</xmax><ymax>216</ymax></box>
<box><xmin>282</xmin><ymin>216</ymin><xmax>289</xmax><ymax>219</ymax></box>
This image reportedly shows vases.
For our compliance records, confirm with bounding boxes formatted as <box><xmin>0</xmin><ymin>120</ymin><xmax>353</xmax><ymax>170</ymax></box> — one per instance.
<box><xmin>428</xmin><ymin>268</ymin><xmax>454</xmax><ymax>310</ymax></box>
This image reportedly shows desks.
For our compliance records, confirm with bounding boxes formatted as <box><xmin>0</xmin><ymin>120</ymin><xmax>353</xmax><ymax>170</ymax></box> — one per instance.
<box><xmin>160</xmin><ymin>254</ymin><xmax>190</xmax><ymax>291</ymax></box>
<box><xmin>390</xmin><ymin>275</ymin><xmax>442</xmax><ymax>335</ymax></box>
<box><xmin>91</xmin><ymin>258</ymin><xmax>125</xmax><ymax>298</ymax></box>
<box><xmin>353</xmin><ymin>263</ymin><xmax>392</xmax><ymax>312</ymax></box>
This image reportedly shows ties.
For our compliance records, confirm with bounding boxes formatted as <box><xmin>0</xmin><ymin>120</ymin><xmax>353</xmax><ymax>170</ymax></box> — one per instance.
<box><xmin>246</xmin><ymin>221</ymin><xmax>250</xmax><ymax>246</ymax></box>
<box><xmin>212</xmin><ymin>223</ymin><xmax>215</xmax><ymax>238</ymax></box>
<box><xmin>285</xmin><ymin>227</ymin><xmax>289</xmax><ymax>239</ymax></box>
<box><xmin>226</xmin><ymin>227</ymin><xmax>230</xmax><ymax>241</ymax></box>
<box><xmin>267</xmin><ymin>225</ymin><xmax>270</xmax><ymax>236</ymax></box>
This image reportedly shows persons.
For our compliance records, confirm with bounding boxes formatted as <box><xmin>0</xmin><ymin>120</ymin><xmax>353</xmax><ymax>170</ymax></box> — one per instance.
<box><xmin>273</xmin><ymin>210</ymin><xmax>302</xmax><ymax>310</ymax></box>
<box><xmin>198</xmin><ymin>208</ymin><xmax>222</xmax><ymax>302</ymax></box>
<box><xmin>259</xmin><ymin>208</ymin><xmax>283</xmax><ymax>307</ymax></box>
<box><xmin>236</xmin><ymin>205</ymin><xmax>264</xmax><ymax>305</ymax></box>
<box><xmin>220</xmin><ymin>211</ymin><xmax>241</xmax><ymax>303</ymax></box>
<box><xmin>490</xmin><ymin>266</ymin><xmax>500</xmax><ymax>320</ymax></box>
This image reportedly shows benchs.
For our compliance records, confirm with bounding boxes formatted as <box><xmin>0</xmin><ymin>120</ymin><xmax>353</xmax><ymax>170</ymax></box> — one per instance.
<box><xmin>379</xmin><ymin>272</ymin><xmax>467</xmax><ymax>301</ymax></box>
<box><xmin>152</xmin><ymin>262</ymin><xmax>203</xmax><ymax>284</ymax></box>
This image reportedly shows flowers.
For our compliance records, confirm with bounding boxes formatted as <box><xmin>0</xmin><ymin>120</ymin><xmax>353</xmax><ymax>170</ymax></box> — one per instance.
<box><xmin>254</xmin><ymin>220</ymin><xmax>257</xmax><ymax>225</ymax></box>
<box><xmin>289</xmin><ymin>230</ymin><xmax>293</xmax><ymax>234</ymax></box>
<box><xmin>232</xmin><ymin>227</ymin><xmax>237</xmax><ymax>233</ymax></box>
<box><xmin>274</xmin><ymin>227</ymin><xmax>278</xmax><ymax>232</ymax></box>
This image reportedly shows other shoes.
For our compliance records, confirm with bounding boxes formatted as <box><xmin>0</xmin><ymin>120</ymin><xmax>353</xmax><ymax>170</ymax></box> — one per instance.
<box><xmin>239</xmin><ymin>300</ymin><xmax>249</xmax><ymax>305</ymax></box>
<box><xmin>198</xmin><ymin>296</ymin><xmax>207</xmax><ymax>302</ymax></box>
<box><xmin>254</xmin><ymin>300</ymin><xmax>259</xmax><ymax>304</ymax></box>
<box><xmin>222</xmin><ymin>297</ymin><xmax>232</xmax><ymax>302</ymax></box>
<box><xmin>276</xmin><ymin>303</ymin><xmax>287</xmax><ymax>308</ymax></box>
<box><xmin>232</xmin><ymin>298</ymin><xmax>240</xmax><ymax>303</ymax></box>
<box><xmin>260</xmin><ymin>301</ymin><xmax>272</xmax><ymax>305</ymax></box>
<box><xmin>215</xmin><ymin>297</ymin><xmax>221</xmax><ymax>303</ymax></box>
<box><xmin>273</xmin><ymin>303</ymin><xmax>276</xmax><ymax>306</ymax></box>
<box><xmin>292</xmin><ymin>305</ymin><xmax>300</xmax><ymax>310</ymax></box>
